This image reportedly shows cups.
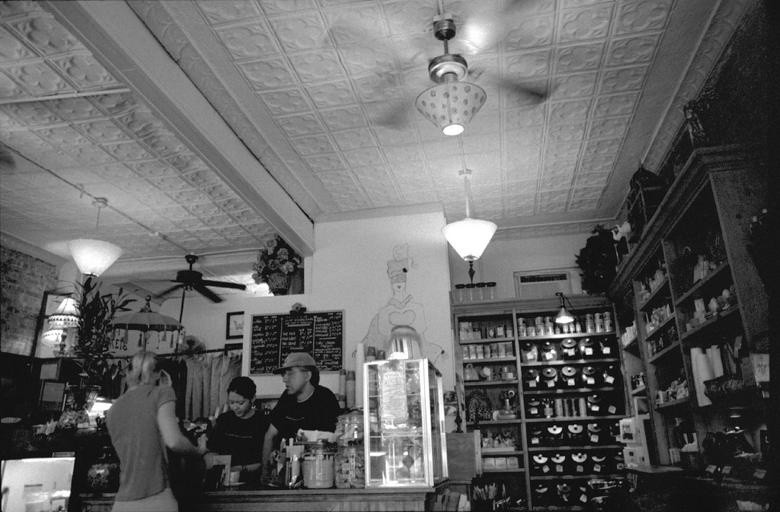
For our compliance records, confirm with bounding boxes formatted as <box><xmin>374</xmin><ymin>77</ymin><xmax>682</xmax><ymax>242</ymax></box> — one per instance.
<box><xmin>518</xmin><ymin>310</ymin><xmax>614</xmax><ymax>335</ymax></box>
<box><xmin>689</xmin><ymin>344</ymin><xmax>725</xmax><ymax>407</ymax></box>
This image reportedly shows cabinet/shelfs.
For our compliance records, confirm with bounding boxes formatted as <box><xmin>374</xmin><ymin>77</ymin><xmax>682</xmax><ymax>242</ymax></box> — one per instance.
<box><xmin>451</xmin><ymin>295</ymin><xmax>632</xmax><ymax>512</ymax></box>
<box><xmin>608</xmin><ymin>138</ymin><xmax>780</xmax><ymax>465</ymax></box>
<box><xmin>363</xmin><ymin>358</ymin><xmax>448</xmax><ymax>488</ymax></box>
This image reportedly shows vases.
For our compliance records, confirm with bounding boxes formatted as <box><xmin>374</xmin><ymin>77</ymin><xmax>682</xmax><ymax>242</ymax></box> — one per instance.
<box><xmin>266</xmin><ymin>272</ymin><xmax>289</xmax><ymax>295</ymax></box>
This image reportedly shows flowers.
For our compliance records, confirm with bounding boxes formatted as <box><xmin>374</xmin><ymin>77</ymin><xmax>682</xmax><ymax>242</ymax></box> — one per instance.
<box><xmin>252</xmin><ymin>238</ymin><xmax>300</xmax><ymax>284</ymax></box>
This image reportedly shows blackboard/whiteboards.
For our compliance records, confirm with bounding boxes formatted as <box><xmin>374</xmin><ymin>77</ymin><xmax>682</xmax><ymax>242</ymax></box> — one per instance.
<box><xmin>249</xmin><ymin>309</ymin><xmax>345</xmax><ymax>377</ymax></box>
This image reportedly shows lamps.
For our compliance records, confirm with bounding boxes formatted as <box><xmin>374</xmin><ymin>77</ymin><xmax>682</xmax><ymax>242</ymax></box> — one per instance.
<box><xmin>556</xmin><ymin>292</ymin><xmax>574</xmax><ymax>324</ymax></box>
<box><xmin>48</xmin><ymin>297</ymin><xmax>83</xmax><ymax>356</ymax></box>
<box><xmin>441</xmin><ymin>170</ymin><xmax>499</xmax><ymax>285</ymax></box>
<box><xmin>416</xmin><ymin>72</ymin><xmax>487</xmax><ymax>136</ymax></box>
<box><xmin>67</xmin><ymin>201</ymin><xmax>125</xmax><ymax>277</ymax></box>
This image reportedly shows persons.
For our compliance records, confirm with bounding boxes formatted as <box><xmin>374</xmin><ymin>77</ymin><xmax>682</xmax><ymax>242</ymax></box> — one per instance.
<box><xmin>261</xmin><ymin>352</ymin><xmax>341</xmax><ymax>488</ymax></box>
<box><xmin>198</xmin><ymin>376</ymin><xmax>275</xmax><ymax>489</ymax></box>
<box><xmin>176</xmin><ymin>410</ymin><xmax>214</xmax><ymax>450</ymax></box>
<box><xmin>103</xmin><ymin>350</ymin><xmax>199</xmax><ymax>512</ymax></box>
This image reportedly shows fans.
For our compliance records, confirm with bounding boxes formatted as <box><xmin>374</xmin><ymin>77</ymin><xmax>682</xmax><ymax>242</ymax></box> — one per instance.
<box><xmin>323</xmin><ymin>0</ymin><xmax>554</xmax><ymax>129</ymax></box>
<box><xmin>138</xmin><ymin>255</ymin><xmax>246</xmax><ymax>303</ymax></box>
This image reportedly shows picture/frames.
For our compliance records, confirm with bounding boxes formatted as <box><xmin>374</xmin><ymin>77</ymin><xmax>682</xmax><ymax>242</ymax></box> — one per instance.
<box><xmin>226</xmin><ymin>311</ymin><xmax>244</xmax><ymax>339</ymax></box>
<box><xmin>224</xmin><ymin>343</ymin><xmax>243</xmax><ymax>356</ymax></box>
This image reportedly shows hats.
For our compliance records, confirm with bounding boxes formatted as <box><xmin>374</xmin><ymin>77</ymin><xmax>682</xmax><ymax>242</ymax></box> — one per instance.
<box><xmin>272</xmin><ymin>351</ymin><xmax>316</xmax><ymax>374</ymax></box>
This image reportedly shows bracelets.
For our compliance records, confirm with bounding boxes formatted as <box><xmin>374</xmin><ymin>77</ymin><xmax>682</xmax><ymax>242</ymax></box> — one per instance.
<box><xmin>241</xmin><ymin>464</ymin><xmax>246</xmax><ymax>472</ymax></box>
<box><xmin>201</xmin><ymin>451</ymin><xmax>210</xmax><ymax>459</ymax></box>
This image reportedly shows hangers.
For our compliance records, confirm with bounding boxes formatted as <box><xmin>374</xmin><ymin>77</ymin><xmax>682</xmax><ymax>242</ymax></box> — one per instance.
<box><xmin>191</xmin><ymin>349</ymin><xmax>242</xmax><ymax>369</ymax></box>
